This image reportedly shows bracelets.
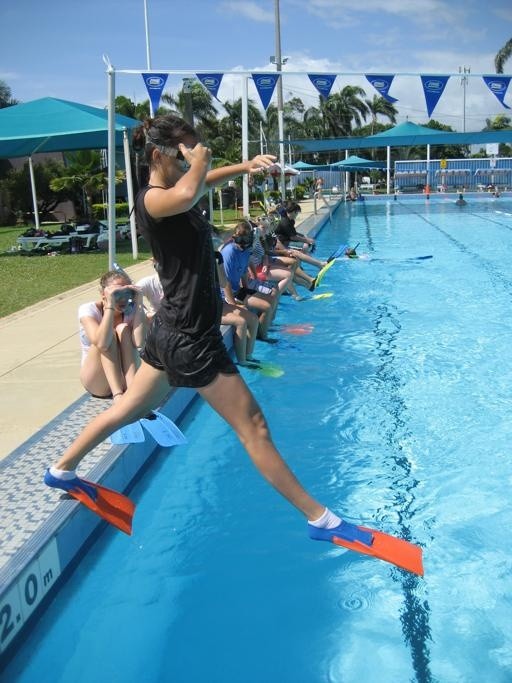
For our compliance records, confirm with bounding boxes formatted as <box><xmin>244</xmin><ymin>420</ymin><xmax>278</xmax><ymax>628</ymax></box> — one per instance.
<box><xmin>103</xmin><ymin>307</ymin><xmax>116</xmax><ymax>311</ymax></box>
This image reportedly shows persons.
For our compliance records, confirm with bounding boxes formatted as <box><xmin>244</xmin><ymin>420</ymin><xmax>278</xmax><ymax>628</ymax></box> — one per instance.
<box><xmin>423</xmin><ymin>183</ymin><xmax>431</xmax><ymax>199</ymax></box>
<box><xmin>39</xmin><ymin>112</ymin><xmax>373</xmax><ymax>548</ymax></box>
<box><xmin>206</xmin><ymin>199</ymin><xmax>337</xmax><ymax>372</ymax></box>
<box><xmin>343</xmin><ymin>246</ymin><xmax>367</xmax><ymax>262</ymax></box>
<box><xmin>456</xmin><ymin>193</ymin><xmax>465</xmax><ymax>203</ymax></box>
<box><xmin>134</xmin><ymin>273</ymin><xmax>165</xmax><ymax>342</ymax></box>
<box><xmin>76</xmin><ymin>269</ymin><xmax>159</xmax><ymax>423</ymax></box>
<box><xmin>315</xmin><ymin>176</ymin><xmax>324</xmax><ymax>199</ymax></box>
<box><xmin>350</xmin><ymin>186</ymin><xmax>357</xmax><ymax>201</ymax></box>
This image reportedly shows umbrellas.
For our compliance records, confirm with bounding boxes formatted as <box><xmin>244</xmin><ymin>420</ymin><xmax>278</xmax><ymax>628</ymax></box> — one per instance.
<box><xmin>291</xmin><ymin>160</ymin><xmax>330</xmax><ymax>172</ymax></box>
<box><xmin>258</xmin><ymin>161</ymin><xmax>301</xmax><ymax>176</ymax></box>
<box><xmin>329</xmin><ymin>154</ymin><xmax>385</xmax><ymax>186</ymax></box>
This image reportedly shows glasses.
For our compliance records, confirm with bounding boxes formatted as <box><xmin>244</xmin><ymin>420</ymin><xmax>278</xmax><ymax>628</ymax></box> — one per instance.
<box><xmin>113</xmin><ymin>289</ymin><xmax>131</xmax><ymax>300</ymax></box>
<box><xmin>230</xmin><ymin>205</ymin><xmax>294</xmax><ymax>252</ymax></box>
<box><xmin>180</xmin><ymin>149</ymin><xmax>199</xmax><ymax>171</ymax></box>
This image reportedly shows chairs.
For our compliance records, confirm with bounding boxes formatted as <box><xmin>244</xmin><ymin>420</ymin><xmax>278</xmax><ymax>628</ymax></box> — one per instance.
<box><xmin>17</xmin><ymin>218</ymin><xmax>138</xmax><ymax>252</ymax></box>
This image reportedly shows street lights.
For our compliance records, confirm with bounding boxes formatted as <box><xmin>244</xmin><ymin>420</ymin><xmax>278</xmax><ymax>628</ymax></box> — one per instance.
<box><xmin>268</xmin><ymin>0</ymin><xmax>293</xmax><ymax>205</ymax></box>
<box><xmin>457</xmin><ymin>65</ymin><xmax>474</xmax><ymax>133</ymax></box>
